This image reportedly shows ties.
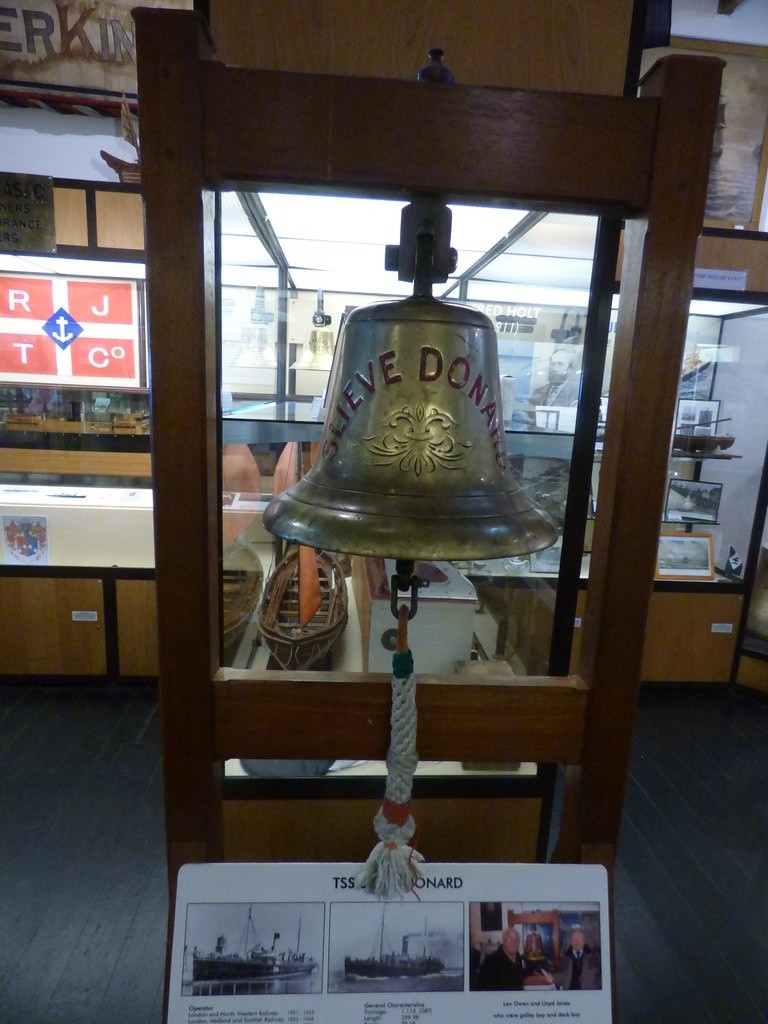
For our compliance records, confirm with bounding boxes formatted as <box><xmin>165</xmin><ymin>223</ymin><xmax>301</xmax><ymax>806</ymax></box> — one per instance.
<box><xmin>576</xmin><ymin>951</ymin><xmax>580</xmax><ymax>961</ymax></box>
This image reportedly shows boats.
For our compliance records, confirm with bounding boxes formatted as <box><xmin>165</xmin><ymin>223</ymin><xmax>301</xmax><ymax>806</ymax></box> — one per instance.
<box><xmin>257</xmin><ymin>544</ymin><xmax>349</xmax><ymax>659</ymax></box>
<box><xmin>193</xmin><ymin>902</ymin><xmax>319</xmax><ymax>981</ymax></box>
<box><xmin>343</xmin><ymin>903</ymin><xmax>447</xmax><ymax>975</ymax></box>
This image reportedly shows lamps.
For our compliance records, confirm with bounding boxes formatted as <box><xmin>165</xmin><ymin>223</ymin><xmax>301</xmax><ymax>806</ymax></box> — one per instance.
<box><xmin>312</xmin><ymin>289</ymin><xmax>332</xmax><ymax>327</ymax></box>
<box><xmin>252</xmin><ymin>286</ymin><xmax>276</xmax><ymax>325</ymax></box>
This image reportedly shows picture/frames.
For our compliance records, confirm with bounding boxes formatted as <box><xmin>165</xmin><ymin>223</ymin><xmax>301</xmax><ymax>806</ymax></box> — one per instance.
<box><xmin>654</xmin><ymin>531</ymin><xmax>715</xmax><ymax>581</ymax></box>
<box><xmin>528</xmin><ymin>534</ymin><xmax>563</xmax><ymax>573</ymax></box>
<box><xmin>675</xmin><ymin>397</ymin><xmax>721</xmax><ymax>435</ymax></box>
<box><xmin>638</xmin><ymin>36</ymin><xmax>768</xmax><ymax>231</ymax></box>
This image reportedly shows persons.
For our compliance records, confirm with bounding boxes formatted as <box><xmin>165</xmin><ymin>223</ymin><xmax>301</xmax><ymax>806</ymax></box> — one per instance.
<box><xmin>478</xmin><ymin>927</ymin><xmax>530</xmax><ymax>991</ymax></box>
<box><xmin>544</xmin><ymin>928</ymin><xmax>601</xmax><ymax>992</ymax></box>
<box><xmin>527</xmin><ymin>349</ymin><xmax>578</xmax><ymax>425</ymax></box>
